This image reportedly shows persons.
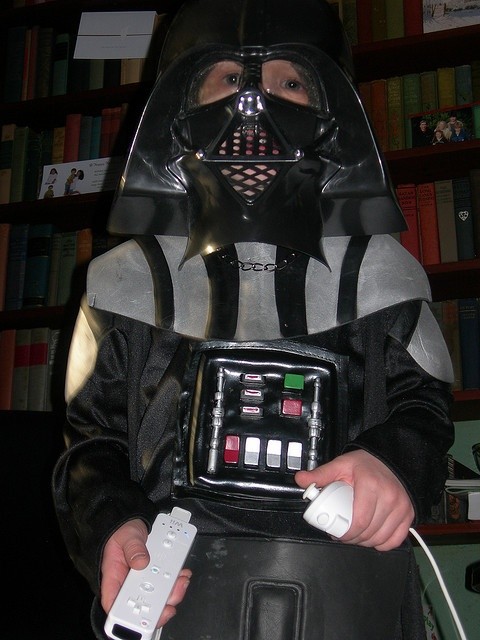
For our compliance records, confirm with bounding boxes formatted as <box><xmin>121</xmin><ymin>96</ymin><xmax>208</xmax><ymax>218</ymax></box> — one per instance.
<box><xmin>45</xmin><ymin>168</ymin><xmax>85</xmax><ymax>197</ymax></box>
<box><xmin>419</xmin><ymin>116</ymin><xmax>466</xmax><ymax>147</ymax></box>
<box><xmin>45</xmin><ymin>1</ymin><xmax>456</xmax><ymax>640</ymax></box>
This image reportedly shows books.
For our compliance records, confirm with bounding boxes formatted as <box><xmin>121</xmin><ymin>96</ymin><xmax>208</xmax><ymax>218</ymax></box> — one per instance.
<box><xmin>392</xmin><ymin>171</ymin><xmax>479</xmax><ymax>265</ymax></box>
<box><xmin>2</xmin><ymin>8</ymin><xmax>155</xmax><ymax>102</ymax></box>
<box><xmin>2</xmin><ymin>224</ymin><xmax>118</xmax><ymax>312</ymax></box>
<box><xmin>431</xmin><ymin>296</ymin><xmax>479</xmax><ymax>392</ymax></box>
<box><xmin>0</xmin><ymin>105</ymin><xmax>131</xmax><ymax>207</ymax></box>
<box><xmin>0</xmin><ymin>329</ymin><xmax>59</xmax><ymax>412</ymax></box>
<box><xmin>356</xmin><ymin>65</ymin><xmax>479</xmax><ymax>152</ymax></box>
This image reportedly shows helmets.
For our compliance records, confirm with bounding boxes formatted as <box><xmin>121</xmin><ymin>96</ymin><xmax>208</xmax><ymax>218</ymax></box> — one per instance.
<box><xmin>107</xmin><ymin>0</ymin><xmax>409</xmax><ymax>271</ymax></box>
